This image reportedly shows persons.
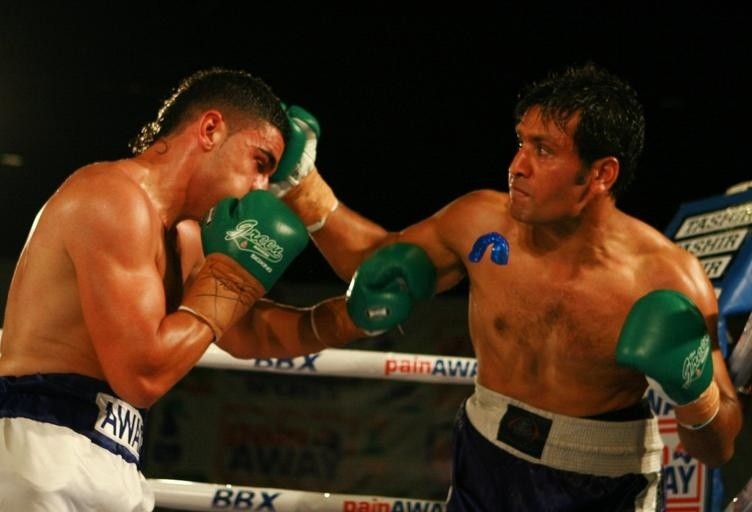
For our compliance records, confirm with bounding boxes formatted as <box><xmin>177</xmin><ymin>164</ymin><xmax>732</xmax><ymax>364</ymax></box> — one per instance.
<box><xmin>0</xmin><ymin>68</ymin><xmax>438</xmax><ymax>512</ymax></box>
<box><xmin>268</xmin><ymin>63</ymin><xmax>744</xmax><ymax>512</ymax></box>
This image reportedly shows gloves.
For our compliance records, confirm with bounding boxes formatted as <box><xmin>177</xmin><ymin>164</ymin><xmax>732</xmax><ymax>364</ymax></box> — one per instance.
<box><xmin>310</xmin><ymin>241</ymin><xmax>436</xmax><ymax>348</ymax></box>
<box><xmin>268</xmin><ymin>102</ymin><xmax>339</xmax><ymax>233</ymax></box>
<box><xmin>174</xmin><ymin>191</ymin><xmax>312</xmax><ymax>345</ymax></box>
<box><xmin>614</xmin><ymin>288</ymin><xmax>721</xmax><ymax>432</ymax></box>
